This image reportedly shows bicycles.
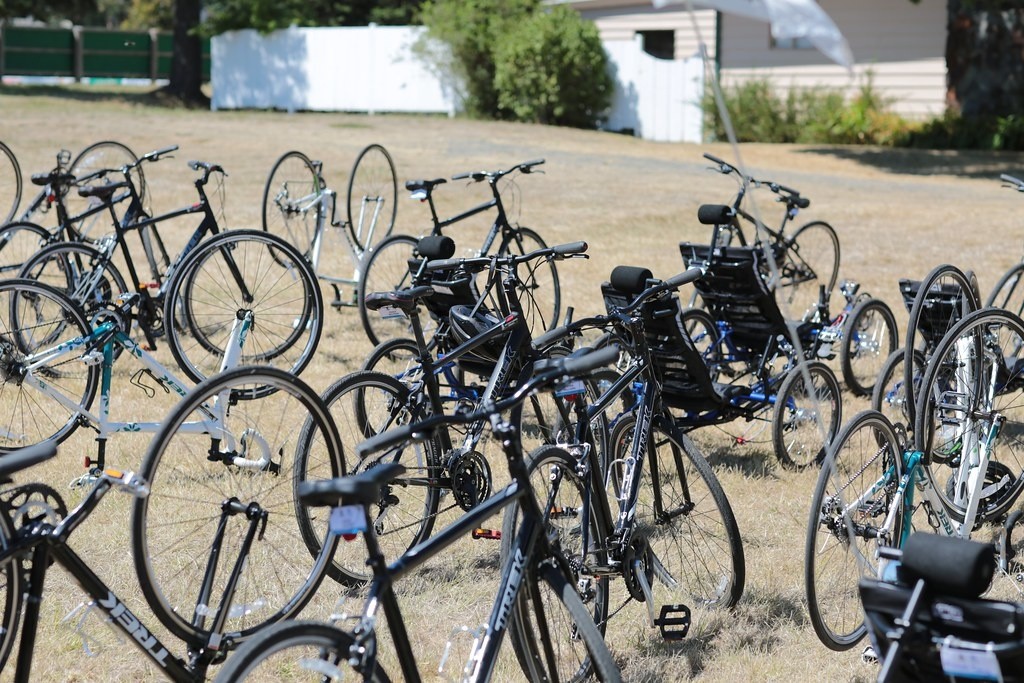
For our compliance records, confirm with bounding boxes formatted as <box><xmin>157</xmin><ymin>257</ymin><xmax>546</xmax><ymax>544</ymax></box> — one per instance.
<box><xmin>0</xmin><ymin>138</ymin><xmax>1022</xmax><ymax>683</ymax></box>
